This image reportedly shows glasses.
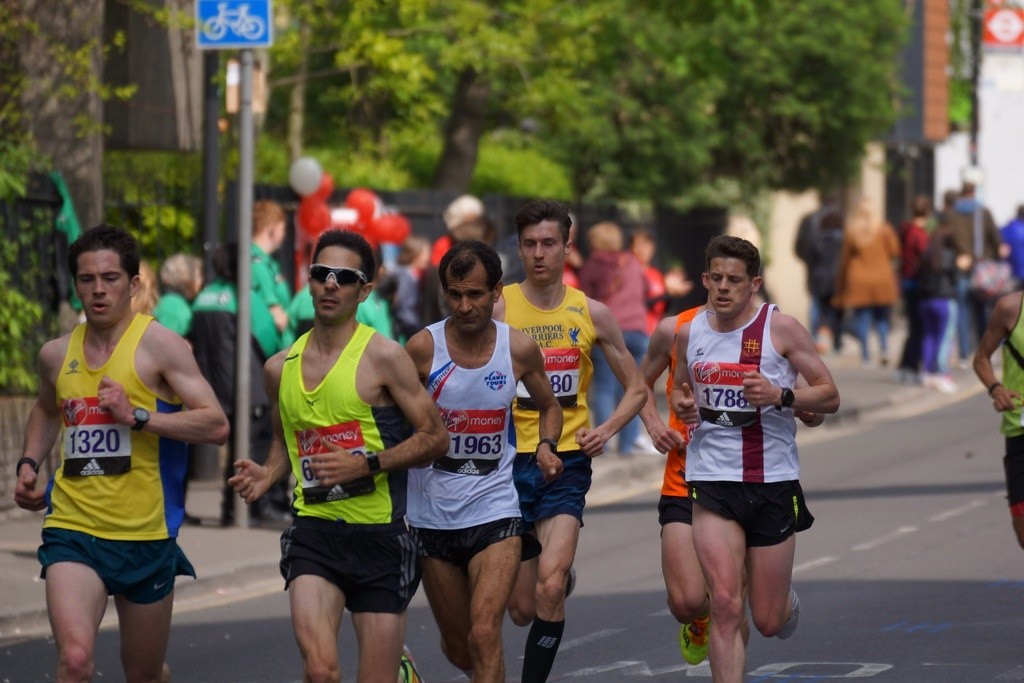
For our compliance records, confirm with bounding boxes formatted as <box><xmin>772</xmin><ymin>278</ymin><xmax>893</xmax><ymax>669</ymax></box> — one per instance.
<box><xmin>309</xmin><ymin>264</ymin><xmax>372</xmax><ymax>285</ymax></box>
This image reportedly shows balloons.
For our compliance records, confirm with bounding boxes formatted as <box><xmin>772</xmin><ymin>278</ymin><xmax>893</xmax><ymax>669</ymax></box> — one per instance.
<box><xmin>290</xmin><ymin>156</ymin><xmax>412</xmax><ymax>254</ymax></box>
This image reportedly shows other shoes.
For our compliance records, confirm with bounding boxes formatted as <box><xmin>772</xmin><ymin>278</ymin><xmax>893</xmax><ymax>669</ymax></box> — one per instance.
<box><xmin>919</xmin><ymin>370</ymin><xmax>961</xmax><ymax>396</ymax></box>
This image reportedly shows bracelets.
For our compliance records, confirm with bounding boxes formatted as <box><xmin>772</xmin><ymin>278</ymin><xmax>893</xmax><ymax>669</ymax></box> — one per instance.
<box><xmin>799</xmin><ymin>413</ymin><xmax>816</xmax><ymax>423</ymax></box>
<box><xmin>16</xmin><ymin>458</ymin><xmax>39</xmax><ymax>476</ymax></box>
<box><xmin>988</xmin><ymin>382</ymin><xmax>1002</xmax><ymax>397</ymax></box>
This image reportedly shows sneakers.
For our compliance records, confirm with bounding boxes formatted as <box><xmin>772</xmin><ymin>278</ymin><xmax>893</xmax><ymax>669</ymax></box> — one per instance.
<box><xmin>777</xmin><ymin>587</ymin><xmax>800</xmax><ymax>640</ymax></box>
<box><xmin>680</xmin><ymin>591</ymin><xmax>711</xmax><ymax>665</ymax></box>
<box><xmin>396</xmin><ymin>645</ymin><xmax>424</xmax><ymax>683</ymax></box>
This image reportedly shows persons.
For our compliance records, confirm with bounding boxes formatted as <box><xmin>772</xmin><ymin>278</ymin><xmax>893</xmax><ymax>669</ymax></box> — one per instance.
<box><xmin>491</xmin><ymin>198</ymin><xmax>648</xmax><ymax>683</ymax></box>
<box><xmin>637</xmin><ymin>234</ymin><xmax>841</xmax><ymax>683</ymax></box>
<box><xmin>77</xmin><ymin>195</ymin><xmax>773</xmax><ymax>527</ymax></box>
<box><xmin>973</xmin><ymin>291</ymin><xmax>1024</xmax><ymax>549</ymax></box>
<box><xmin>795</xmin><ymin>182</ymin><xmax>1024</xmax><ymax>394</ymax></box>
<box><xmin>404</xmin><ymin>240</ymin><xmax>565</xmax><ymax>683</ymax></box>
<box><xmin>228</xmin><ymin>229</ymin><xmax>450</xmax><ymax>683</ymax></box>
<box><xmin>14</xmin><ymin>223</ymin><xmax>229</xmax><ymax>683</ymax></box>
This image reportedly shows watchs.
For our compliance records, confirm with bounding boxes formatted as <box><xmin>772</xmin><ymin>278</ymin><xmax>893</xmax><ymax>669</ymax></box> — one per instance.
<box><xmin>535</xmin><ymin>438</ymin><xmax>558</xmax><ymax>454</ymax></box>
<box><xmin>130</xmin><ymin>407</ymin><xmax>150</xmax><ymax>432</ymax></box>
<box><xmin>363</xmin><ymin>451</ymin><xmax>381</xmax><ymax>476</ymax></box>
<box><xmin>775</xmin><ymin>387</ymin><xmax>794</xmax><ymax>411</ymax></box>
